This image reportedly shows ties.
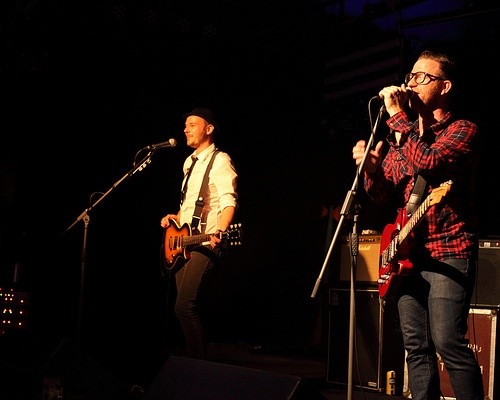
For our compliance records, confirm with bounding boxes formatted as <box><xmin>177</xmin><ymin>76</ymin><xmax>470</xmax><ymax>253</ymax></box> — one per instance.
<box><xmin>180</xmin><ymin>157</ymin><xmax>198</xmax><ymax>206</ymax></box>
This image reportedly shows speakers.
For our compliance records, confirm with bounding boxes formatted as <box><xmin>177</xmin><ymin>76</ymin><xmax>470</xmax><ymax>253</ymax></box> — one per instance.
<box><xmin>325</xmin><ymin>234</ymin><xmax>500</xmax><ymax>394</ymax></box>
<box><xmin>143</xmin><ymin>356</ymin><xmax>313</xmax><ymax>400</ymax></box>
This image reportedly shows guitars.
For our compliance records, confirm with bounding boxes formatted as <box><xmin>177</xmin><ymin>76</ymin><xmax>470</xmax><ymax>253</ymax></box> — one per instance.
<box><xmin>161</xmin><ymin>219</ymin><xmax>247</xmax><ymax>273</ymax></box>
<box><xmin>377</xmin><ymin>179</ymin><xmax>453</xmax><ymax>300</ymax></box>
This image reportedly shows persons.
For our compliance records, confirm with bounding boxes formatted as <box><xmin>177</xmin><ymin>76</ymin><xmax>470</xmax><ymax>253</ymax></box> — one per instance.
<box><xmin>161</xmin><ymin>108</ymin><xmax>240</xmax><ymax>360</ymax></box>
<box><xmin>353</xmin><ymin>50</ymin><xmax>484</xmax><ymax>400</ymax></box>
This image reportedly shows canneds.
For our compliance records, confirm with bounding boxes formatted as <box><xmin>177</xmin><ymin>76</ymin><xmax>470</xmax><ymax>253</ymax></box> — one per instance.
<box><xmin>386</xmin><ymin>370</ymin><xmax>396</xmax><ymax>395</ymax></box>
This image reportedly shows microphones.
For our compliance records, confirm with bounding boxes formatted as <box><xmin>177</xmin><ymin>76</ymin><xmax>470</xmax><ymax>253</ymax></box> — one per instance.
<box><xmin>374</xmin><ymin>87</ymin><xmax>414</xmax><ymax>100</ymax></box>
<box><xmin>146</xmin><ymin>139</ymin><xmax>177</xmax><ymax>149</ymax></box>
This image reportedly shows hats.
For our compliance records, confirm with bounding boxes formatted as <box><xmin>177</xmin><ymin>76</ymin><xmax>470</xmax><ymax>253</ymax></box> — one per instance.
<box><xmin>183</xmin><ymin>108</ymin><xmax>218</xmax><ymax>129</ymax></box>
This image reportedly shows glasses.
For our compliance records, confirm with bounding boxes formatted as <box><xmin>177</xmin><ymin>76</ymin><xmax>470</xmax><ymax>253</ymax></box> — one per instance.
<box><xmin>404</xmin><ymin>71</ymin><xmax>446</xmax><ymax>85</ymax></box>
